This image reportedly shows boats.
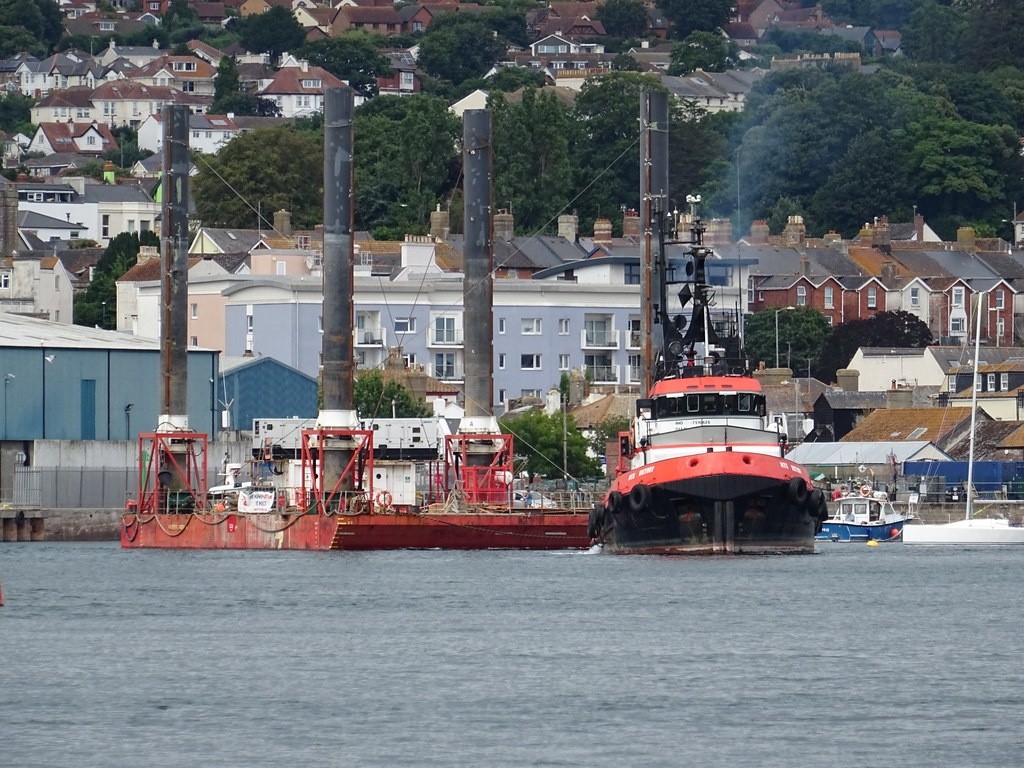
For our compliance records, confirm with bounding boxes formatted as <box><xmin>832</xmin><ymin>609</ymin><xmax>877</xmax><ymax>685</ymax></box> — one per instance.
<box><xmin>587</xmin><ymin>188</ymin><xmax>827</xmax><ymax>566</ymax></box>
<box><xmin>113</xmin><ymin>85</ymin><xmax>675</xmax><ymax>552</ymax></box>
<box><xmin>830</xmin><ymin>488</ymin><xmax>911</xmax><ymax>546</ymax></box>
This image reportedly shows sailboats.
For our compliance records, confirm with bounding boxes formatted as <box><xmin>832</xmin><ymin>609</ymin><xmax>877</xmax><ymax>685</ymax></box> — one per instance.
<box><xmin>899</xmin><ymin>291</ymin><xmax>1024</xmax><ymax>546</ymax></box>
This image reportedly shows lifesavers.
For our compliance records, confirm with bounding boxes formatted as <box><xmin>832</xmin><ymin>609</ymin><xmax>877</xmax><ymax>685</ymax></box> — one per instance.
<box><xmin>375</xmin><ymin>490</ymin><xmax>392</xmax><ymax>507</ymax></box>
<box><xmin>860</xmin><ymin>485</ymin><xmax>870</xmax><ymax>497</ymax></box>
